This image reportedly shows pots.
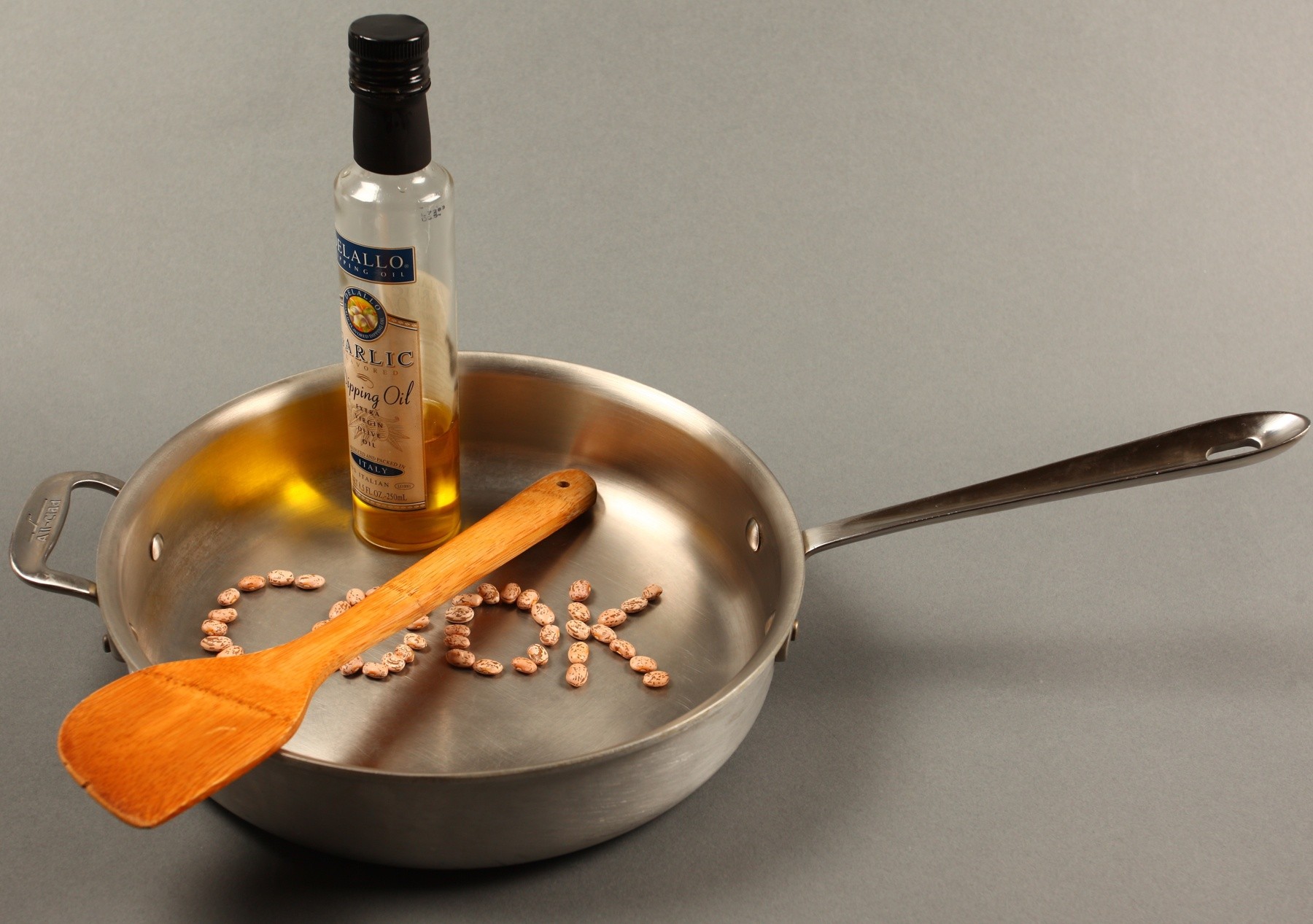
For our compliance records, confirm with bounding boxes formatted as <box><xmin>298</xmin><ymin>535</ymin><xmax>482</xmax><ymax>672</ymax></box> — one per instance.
<box><xmin>9</xmin><ymin>351</ymin><xmax>1309</xmax><ymax>870</ymax></box>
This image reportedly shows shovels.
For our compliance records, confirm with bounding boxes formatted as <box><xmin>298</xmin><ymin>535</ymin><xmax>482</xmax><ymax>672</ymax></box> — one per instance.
<box><xmin>58</xmin><ymin>469</ymin><xmax>597</xmax><ymax>830</ymax></box>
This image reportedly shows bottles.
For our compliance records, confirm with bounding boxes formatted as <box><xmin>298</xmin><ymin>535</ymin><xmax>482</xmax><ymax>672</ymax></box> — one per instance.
<box><xmin>332</xmin><ymin>13</ymin><xmax>463</xmax><ymax>554</ymax></box>
